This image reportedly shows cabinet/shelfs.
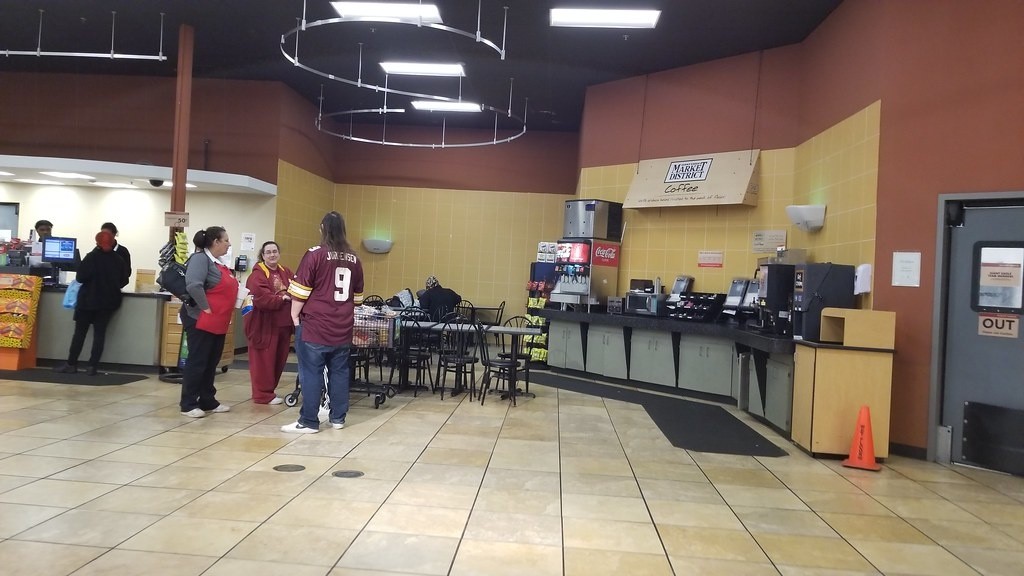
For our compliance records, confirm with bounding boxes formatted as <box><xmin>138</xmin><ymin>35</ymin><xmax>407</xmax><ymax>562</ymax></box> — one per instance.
<box><xmin>666</xmin><ymin>292</ymin><xmax>727</xmax><ymax>322</ymax></box>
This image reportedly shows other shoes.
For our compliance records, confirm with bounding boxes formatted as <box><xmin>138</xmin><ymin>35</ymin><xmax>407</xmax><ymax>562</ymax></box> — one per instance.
<box><xmin>54</xmin><ymin>361</ymin><xmax>77</xmax><ymax>374</ymax></box>
<box><xmin>268</xmin><ymin>398</ymin><xmax>283</xmax><ymax>404</ymax></box>
<box><xmin>204</xmin><ymin>404</ymin><xmax>230</xmax><ymax>413</ymax></box>
<box><xmin>86</xmin><ymin>367</ymin><xmax>97</xmax><ymax>376</ymax></box>
<box><xmin>180</xmin><ymin>408</ymin><xmax>205</xmax><ymax>417</ymax></box>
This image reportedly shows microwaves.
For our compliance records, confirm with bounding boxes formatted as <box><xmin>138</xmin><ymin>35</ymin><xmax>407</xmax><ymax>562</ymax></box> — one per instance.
<box><xmin>626</xmin><ymin>290</ymin><xmax>669</xmax><ymax>317</ymax></box>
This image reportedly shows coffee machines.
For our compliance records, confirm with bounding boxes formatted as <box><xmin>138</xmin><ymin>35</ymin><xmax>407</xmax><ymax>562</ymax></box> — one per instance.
<box><xmin>748</xmin><ymin>264</ymin><xmax>795</xmax><ymax>328</ymax></box>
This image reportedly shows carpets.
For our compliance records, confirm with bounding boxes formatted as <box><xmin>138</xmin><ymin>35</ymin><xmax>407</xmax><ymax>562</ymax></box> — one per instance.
<box><xmin>0</xmin><ymin>368</ymin><xmax>150</xmax><ymax>386</ymax></box>
<box><xmin>492</xmin><ymin>371</ymin><xmax>789</xmax><ymax>458</ymax></box>
<box><xmin>228</xmin><ymin>360</ymin><xmax>299</xmax><ymax>372</ymax></box>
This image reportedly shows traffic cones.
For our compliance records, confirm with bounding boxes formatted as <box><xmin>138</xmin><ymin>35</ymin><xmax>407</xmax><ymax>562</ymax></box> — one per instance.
<box><xmin>841</xmin><ymin>405</ymin><xmax>882</xmax><ymax>470</ymax></box>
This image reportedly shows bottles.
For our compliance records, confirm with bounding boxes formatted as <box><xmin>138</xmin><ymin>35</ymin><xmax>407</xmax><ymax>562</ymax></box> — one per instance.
<box><xmin>655</xmin><ymin>277</ymin><xmax>661</xmax><ymax>294</ymax></box>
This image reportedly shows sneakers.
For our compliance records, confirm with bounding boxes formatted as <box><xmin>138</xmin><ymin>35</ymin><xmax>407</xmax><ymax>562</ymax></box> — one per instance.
<box><xmin>332</xmin><ymin>423</ymin><xmax>344</xmax><ymax>429</ymax></box>
<box><xmin>280</xmin><ymin>422</ymin><xmax>319</xmax><ymax>434</ymax></box>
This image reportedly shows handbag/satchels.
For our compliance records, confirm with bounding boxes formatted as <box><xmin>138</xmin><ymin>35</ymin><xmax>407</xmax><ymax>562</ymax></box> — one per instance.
<box><xmin>241</xmin><ymin>295</ymin><xmax>254</xmax><ymax>319</ymax></box>
<box><xmin>156</xmin><ymin>252</ymin><xmax>210</xmax><ymax>307</ymax></box>
<box><xmin>63</xmin><ymin>280</ymin><xmax>83</xmax><ymax>308</ymax></box>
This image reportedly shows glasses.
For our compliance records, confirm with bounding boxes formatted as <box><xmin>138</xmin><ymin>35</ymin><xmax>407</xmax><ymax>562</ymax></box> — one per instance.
<box><xmin>263</xmin><ymin>250</ymin><xmax>279</xmax><ymax>255</ymax></box>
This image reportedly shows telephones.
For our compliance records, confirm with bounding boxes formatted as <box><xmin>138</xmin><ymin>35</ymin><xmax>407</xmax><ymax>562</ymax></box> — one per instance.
<box><xmin>235</xmin><ymin>255</ymin><xmax>248</xmax><ymax>272</ymax></box>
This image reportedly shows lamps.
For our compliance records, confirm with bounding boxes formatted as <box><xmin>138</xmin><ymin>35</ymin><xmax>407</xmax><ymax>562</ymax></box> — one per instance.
<box><xmin>787</xmin><ymin>205</ymin><xmax>827</xmax><ymax>233</ymax></box>
<box><xmin>362</xmin><ymin>239</ymin><xmax>393</xmax><ymax>254</ymax></box>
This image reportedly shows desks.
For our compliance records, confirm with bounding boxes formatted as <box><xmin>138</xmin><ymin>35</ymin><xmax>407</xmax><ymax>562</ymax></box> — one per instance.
<box><xmin>382</xmin><ymin>320</ymin><xmax>439</xmax><ymax>391</ymax></box>
<box><xmin>431</xmin><ymin>323</ymin><xmax>491</xmax><ymax>398</ymax></box>
<box><xmin>376</xmin><ymin>305</ymin><xmax>419</xmax><ymax>311</ymax></box>
<box><xmin>393</xmin><ymin>311</ymin><xmax>425</xmax><ymax>317</ymax></box>
<box><xmin>487</xmin><ymin>326</ymin><xmax>542</xmax><ymax>401</ymax></box>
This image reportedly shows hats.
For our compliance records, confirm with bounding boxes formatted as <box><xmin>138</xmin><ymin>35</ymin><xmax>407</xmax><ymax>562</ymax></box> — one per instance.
<box><xmin>426</xmin><ymin>276</ymin><xmax>439</xmax><ymax>290</ymax></box>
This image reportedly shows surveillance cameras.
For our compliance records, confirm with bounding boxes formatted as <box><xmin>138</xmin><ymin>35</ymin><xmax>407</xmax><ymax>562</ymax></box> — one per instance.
<box><xmin>150</xmin><ymin>180</ymin><xmax>164</xmax><ymax>187</ymax></box>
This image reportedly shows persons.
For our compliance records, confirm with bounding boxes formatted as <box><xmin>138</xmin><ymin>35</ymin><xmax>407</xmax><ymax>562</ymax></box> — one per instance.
<box><xmin>280</xmin><ymin>210</ymin><xmax>364</xmax><ymax>434</ymax></box>
<box><xmin>53</xmin><ymin>230</ymin><xmax>130</xmax><ymax>375</ymax></box>
<box><xmin>179</xmin><ymin>227</ymin><xmax>239</xmax><ymax>418</ymax></box>
<box><xmin>36</xmin><ymin>220</ymin><xmax>53</xmax><ymax>242</ymax></box>
<box><xmin>101</xmin><ymin>223</ymin><xmax>132</xmax><ymax>277</ymax></box>
<box><xmin>242</xmin><ymin>241</ymin><xmax>295</xmax><ymax>405</ymax></box>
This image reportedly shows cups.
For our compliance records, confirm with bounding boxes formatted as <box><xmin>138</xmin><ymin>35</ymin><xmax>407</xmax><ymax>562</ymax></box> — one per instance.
<box><xmin>661</xmin><ymin>286</ymin><xmax>666</xmax><ymax>294</ymax></box>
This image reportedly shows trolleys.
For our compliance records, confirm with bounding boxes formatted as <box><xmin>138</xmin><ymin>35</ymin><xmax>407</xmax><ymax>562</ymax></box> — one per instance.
<box><xmin>284</xmin><ymin>299</ymin><xmax>402</xmax><ymax>409</ymax></box>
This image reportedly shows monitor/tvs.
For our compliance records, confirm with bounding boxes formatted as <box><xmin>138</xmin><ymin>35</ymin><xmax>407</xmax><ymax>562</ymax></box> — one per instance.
<box><xmin>9</xmin><ymin>250</ymin><xmax>27</xmax><ymax>266</ymax></box>
<box><xmin>42</xmin><ymin>238</ymin><xmax>81</xmax><ymax>271</ymax></box>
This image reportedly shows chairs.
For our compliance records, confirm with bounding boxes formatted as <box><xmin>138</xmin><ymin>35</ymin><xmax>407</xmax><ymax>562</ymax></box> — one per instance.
<box><xmin>348</xmin><ymin>294</ymin><xmax>534</xmax><ymax>406</ymax></box>
